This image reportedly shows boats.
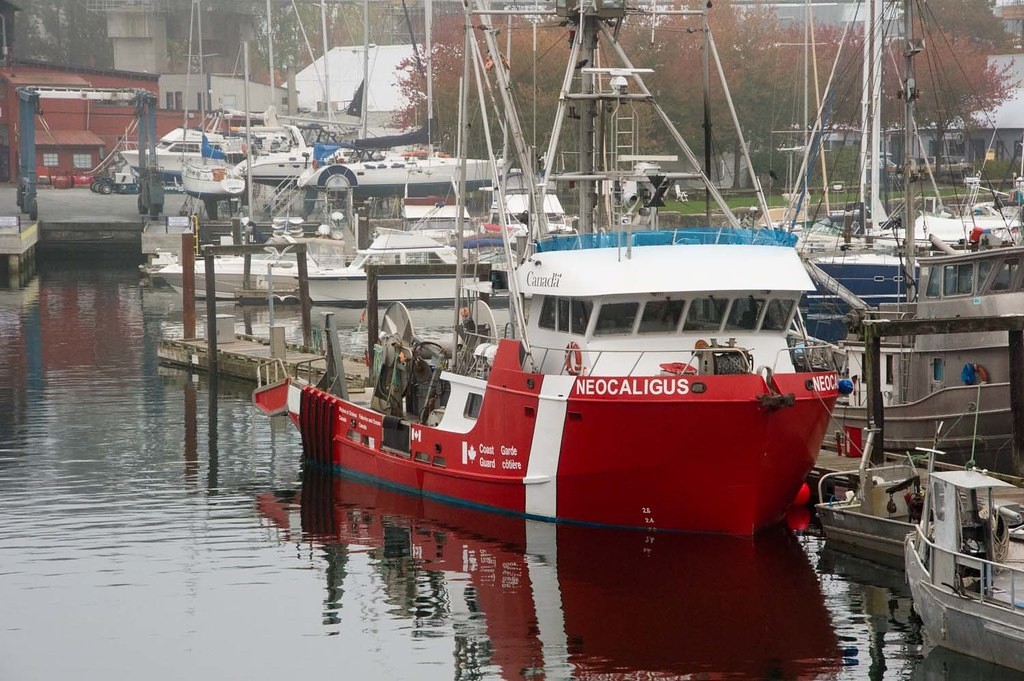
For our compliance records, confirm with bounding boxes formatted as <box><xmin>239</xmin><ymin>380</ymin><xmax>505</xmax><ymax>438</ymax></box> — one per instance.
<box><xmin>754</xmin><ymin>0</ymin><xmax>1024</xmax><ymax>464</ymax></box>
<box><xmin>903</xmin><ymin>387</ymin><xmax>1024</xmax><ymax>673</ymax></box>
<box><xmin>815</xmin><ymin>416</ymin><xmax>933</xmax><ymax>570</ymax></box>
<box><xmin>122</xmin><ymin>123</ymin><xmax>264</xmax><ymax>183</ymax></box>
<box><xmin>156</xmin><ymin>186</ymin><xmax>577</xmax><ymax>307</ymax></box>
<box><xmin>246</xmin><ymin>1</ymin><xmax>847</xmax><ymax>543</ymax></box>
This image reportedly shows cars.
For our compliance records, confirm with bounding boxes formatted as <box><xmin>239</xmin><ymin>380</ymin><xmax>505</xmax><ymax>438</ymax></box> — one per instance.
<box><xmin>866</xmin><ymin>157</ymin><xmax>898</xmax><ymax>169</ymax></box>
<box><xmin>914</xmin><ymin>155</ymin><xmax>974</xmax><ymax>177</ymax></box>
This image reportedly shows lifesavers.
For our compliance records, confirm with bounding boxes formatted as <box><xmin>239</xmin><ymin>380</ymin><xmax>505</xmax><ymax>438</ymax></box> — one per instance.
<box><xmin>965</xmin><ymin>364</ymin><xmax>987</xmax><ymax>385</ymax></box>
<box><xmin>564</xmin><ymin>341</ymin><xmax>582</xmax><ymax>376</ymax></box>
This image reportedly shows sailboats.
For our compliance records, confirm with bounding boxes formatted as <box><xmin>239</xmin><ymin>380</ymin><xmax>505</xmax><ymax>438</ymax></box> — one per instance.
<box><xmin>244</xmin><ymin>1</ymin><xmax>501</xmax><ymax>201</ymax></box>
<box><xmin>181</xmin><ymin>1</ymin><xmax>246</xmax><ymax>198</ymax></box>
